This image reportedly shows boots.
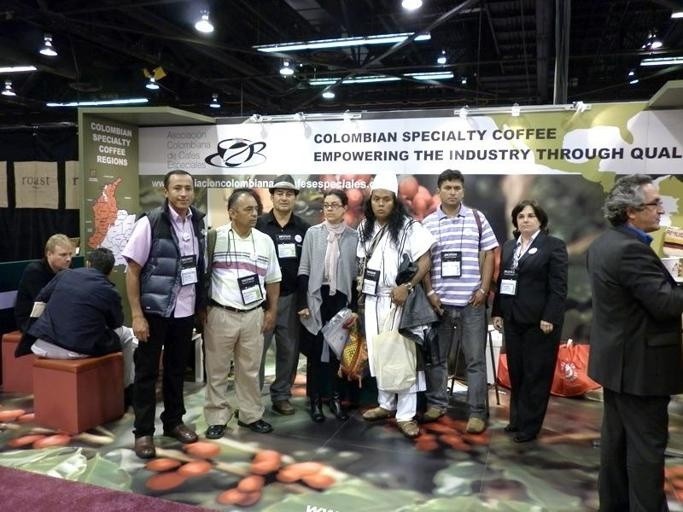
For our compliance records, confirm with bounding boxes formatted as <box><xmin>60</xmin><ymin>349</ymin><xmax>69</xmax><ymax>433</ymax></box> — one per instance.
<box><xmin>327</xmin><ymin>391</ymin><xmax>348</xmax><ymax>421</ymax></box>
<box><xmin>309</xmin><ymin>396</ymin><xmax>326</xmax><ymax>423</ymax></box>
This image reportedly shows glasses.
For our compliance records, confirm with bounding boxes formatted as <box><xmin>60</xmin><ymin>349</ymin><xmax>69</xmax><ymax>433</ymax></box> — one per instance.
<box><xmin>320</xmin><ymin>202</ymin><xmax>344</xmax><ymax>209</ymax></box>
<box><xmin>639</xmin><ymin>199</ymin><xmax>664</xmax><ymax>207</ymax></box>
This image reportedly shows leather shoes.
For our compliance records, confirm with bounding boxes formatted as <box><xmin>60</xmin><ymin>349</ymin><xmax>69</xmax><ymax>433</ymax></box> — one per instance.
<box><xmin>161</xmin><ymin>421</ymin><xmax>199</xmax><ymax>445</ymax></box>
<box><xmin>236</xmin><ymin>419</ymin><xmax>273</xmax><ymax>434</ymax></box>
<box><xmin>513</xmin><ymin>430</ymin><xmax>536</xmax><ymax>442</ymax></box>
<box><xmin>202</xmin><ymin>423</ymin><xmax>227</xmax><ymax>439</ymax></box>
<box><xmin>503</xmin><ymin>421</ymin><xmax>523</xmax><ymax>433</ymax></box>
<box><xmin>133</xmin><ymin>434</ymin><xmax>156</xmax><ymax>459</ymax></box>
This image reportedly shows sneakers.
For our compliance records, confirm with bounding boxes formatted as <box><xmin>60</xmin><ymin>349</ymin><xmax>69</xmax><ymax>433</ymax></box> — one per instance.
<box><xmin>361</xmin><ymin>405</ymin><xmax>396</xmax><ymax>420</ymax></box>
<box><xmin>465</xmin><ymin>417</ymin><xmax>488</xmax><ymax>434</ymax></box>
<box><xmin>271</xmin><ymin>398</ymin><xmax>297</xmax><ymax>416</ymax></box>
<box><xmin>422</xmin><ymin>408</ymin><xmax>446</xmax><ymax>421</ymax></box>
<box><xmin>393</xmin><ymin>420</ymin><xmax>421</xmax><ymax>438</ymax></box>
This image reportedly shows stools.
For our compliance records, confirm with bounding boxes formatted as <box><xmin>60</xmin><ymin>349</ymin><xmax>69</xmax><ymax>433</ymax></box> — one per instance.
<box><xmin>29</xmin><ymin>351</ymin><xmax>128</xmax><ymax>433</ymax></box>
<box><xmin>1</xmin><ymin>329</ymin><xmax>35</xmax><ymax>398</ymax></box>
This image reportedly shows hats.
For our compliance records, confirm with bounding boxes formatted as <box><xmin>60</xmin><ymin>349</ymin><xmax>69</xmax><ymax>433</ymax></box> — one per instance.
<box><xmin>266</xmin><ymin>173</ymin><xmax>300</xmax><ymax>196</ymax></box>
<box><xmin>368</xmin><ymin>160</ymin><xmax>399</xmax><ymax>199</ymax></box>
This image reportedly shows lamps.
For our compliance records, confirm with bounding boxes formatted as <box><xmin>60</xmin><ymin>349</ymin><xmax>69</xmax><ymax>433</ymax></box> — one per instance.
<box><xmin>39</xmin><ymin>33</ymin><xmax>57</xmax><ymax>56</ymax></box>
<box><xmin>279</xmin><ymin>58</ymin><xmax>294</xmax><ymax>76</ymax></box>
<box><xmin>622</xmin><ymin>8</ymin><xmax>682</xmax><ymax>87</ymax></box>
<box><xmin>0</xmin><ymin>65</ymin><xmax>37</xmax><ymax>73</ymax></box>
<box><xmin>1</xmin><ymin>80</ymin><xmax>16</xmax><ymax>96</ymax></box>
<box><xmin>451</xmin><ymin>99</ymin><xmax>592</xmax><ymax>119</ymax></box>
<box><xmin>46</xmin><ymin>98</ymin><xmax>148</xmax><ymax>107</ymax></box>
<box><xmin>193</xmin><ymin>6</ymin><xmax>214</xmax><ymax>33</ymax></box>
<box><xmin>252</xmin><ymin>32</ymin><xmax>431</xmax><ymax>53</ymax></box>
<box><xmin>437</xmin><ymin>50</ymin><xmax>447</xmax><ymax>65</ymax></box>
<box><xmin>309</xmin><ymin>71</ymin><xmax>454</xmax><ymax>85</ymax></box>
<box><xmin>145</xmin><ymin>72</ymin><xmax>159</xmax><ymax>89</ymax></box>
<box><xmin>209</xmin><ymin>94</ymin><xmax>221</xmax><ymax>108</ymax></box>
